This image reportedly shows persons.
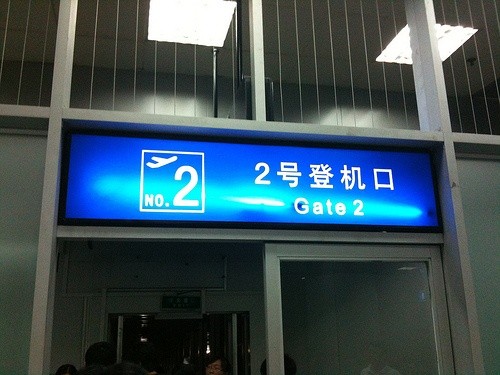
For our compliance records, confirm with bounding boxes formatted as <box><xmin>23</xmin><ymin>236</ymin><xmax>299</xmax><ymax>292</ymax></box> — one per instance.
<box><xmin>204</xmin><ymin>354</ymin><xmax>230</xmax><ymax>375</ymax></box>
<box><xmin>260</xmin><ymin>353</ymin><xmax>297</xmax><ymax>375</ymax></box>
<box><xmin>359</xmin><ymin>340</ymin><xmax>401</xmax><ymax>375</ymax></box>
<box><xmin>170</xmin><ymin>364</ymin><xmax>195</xmax><ymax>375</ymax></box>
<box><xmin>54</xmin><ymin>342</ymin><xmax>156</xmax><ymax>375</ymax></box>
